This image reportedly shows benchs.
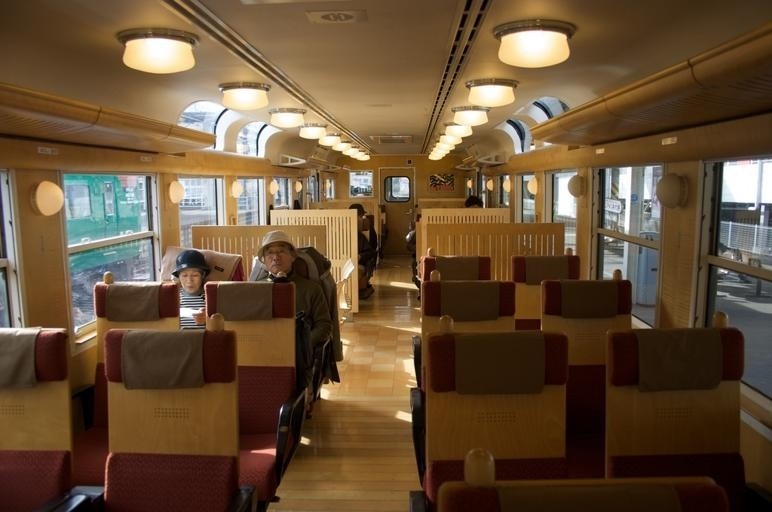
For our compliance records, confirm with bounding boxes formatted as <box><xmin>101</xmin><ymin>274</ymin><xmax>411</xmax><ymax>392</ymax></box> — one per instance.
<box><xmin>412</xmin><ymin>198</ymin><xmax>566</xmax><ymax>291</ymax></box>
<box><xmin>192</xmin><ymin>198</ymin><xmax>381</xmax><ymax>315</ymax></box>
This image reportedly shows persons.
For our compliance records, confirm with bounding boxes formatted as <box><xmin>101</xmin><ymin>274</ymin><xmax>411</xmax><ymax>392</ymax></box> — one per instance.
<box><xmin>253</xmin><ymin>230</ymin><xmax>334</xmax><ymax>388</ymax></box>
<box><xmin>171</xmin><ymin>251</ymin><xmax>212</xmax><ymax>331</ymax></box>
<box><xmin>348</xmin><ymin>204</ymin><xmax>377</xmax><ymax>279</ymax></box>
<box><xmin>465</xmin><ymin>196</ymin><xmax>483</xmax><ymax>208</ymax></box>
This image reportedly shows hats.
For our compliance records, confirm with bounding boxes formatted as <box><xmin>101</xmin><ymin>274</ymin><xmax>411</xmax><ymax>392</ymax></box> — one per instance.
<box><xmin>172</xmin><ymin>250</ymin><xmax>211</xmax><ymax>278</ymax></box>
<box><xmin>258</xmin><ymin>230</ymin><xmax>297</xmax><ymax>265</ymax></box>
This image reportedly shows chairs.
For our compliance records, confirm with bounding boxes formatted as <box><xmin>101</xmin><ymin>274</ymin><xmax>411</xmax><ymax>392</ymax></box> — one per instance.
<box><xmin>0</xmin><ymin>324</ymin><xmax>76</xmax><ymax>512</ymax></box>
<box><xmin>249</xmin><ymin>246</ymin><xmax>344</xmax><ymax>404</ymax></box>
<box><xmin>202</xmin><ymin>280</ymin><xmax>312</xmax><ymax>511</ymax></box>
<box><xmin>67</xmin><ymin>274</ymin><xmax>182</xmax><ymax>500</ymax></box>
<box><xmin>598</xmin><ymin>326</ymin><xmax>757</xmax><ymax>512</ymax></box>
<box><xmin>535</xmin><ymin>271</ymin><xmax>637</xmax><ymax>482</ymax></box>
<box><xmin>158</xmin><ymin>243</ymin><xmax>243</xmax><ymax>286</ymax></box>
<box><xmin>403</xmin><ymin>323</ymin><xmax>595</xmax><ymax>510</ymax></box>
<box><xmin>51</xmin><ymin>324</ymin><xmax>247</xmax><ymax>511</ymax></box>
<box><xmin>410</xmin><ymin>276</ymin><xmax>519</xmax><ymax>395</ymax></box>
<box><xmin>434</xmin><ymin>473</ymin><xmax>731</xmax><ymax>511</ymax></box>
<box><xmin>508</xmin><ymin>247</ymin><xmax>583</xmax><ymax>338</ymax></box>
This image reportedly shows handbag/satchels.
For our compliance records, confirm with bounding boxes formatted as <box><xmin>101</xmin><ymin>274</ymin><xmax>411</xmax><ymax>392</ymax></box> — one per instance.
<box><xmin>296</xmin><ymin>310</ymin><xmax>312</xmax><ymax>389</ymax></box>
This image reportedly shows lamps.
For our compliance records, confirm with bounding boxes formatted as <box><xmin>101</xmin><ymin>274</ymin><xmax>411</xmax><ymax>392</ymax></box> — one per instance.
<box><xmin>264</xmin><ymin>180</ymin><xmax>279</xmax><ymax>199</ymax></box>
<box><xmin>116</xmin><ymin>25</ymin><xmax>196</xmax><ymax>77</ymax></box>
<box><xmin>501</xmin><ymin>179</ymin><xmax>512</xmax><ymax>192</ymax></box>
<box><xmin>490</xmin><ymin>18</ymin><xmax>579</xmax><ymax>71</ymax></box>
<box><xmin>526</xmin><ymin>174</ymin><xmax>539</xmax><ymax>198</ymax></box>
<box><xmin>565</xmin><ymin>172</ymin><xmax>588</xmax><ymax>201</ymax></box>
<box><xmin>424</xmin><ymin>78</ymin><xmax>519</xmax><ymax>164</ymax></box>
<box><xmin>483</xmin><ymin>177</ymin><xmax>497</xmax><ymax>193</ymax></box>
<box><xmin>163</xmin><ymin>179</ymin><xmax>185</xmax><ymax>208</ymax></box>
<box><xmin>654</xmin><ymin>167</ymin><xmax>691</xmax><ymax>212</ymax></box>
<box><xmin>23</xmin><ymin>176</ymin><xmax>70</xmax><ymax>216</ymax></box>
<box><xmin>291</xmin><ymin>179</ymin><xmax>307</xmax><ymax>196</ymax></box>
<box><xmin>266</xmin><ymin>105</ymin><xmax>373</xmax><ymax>164</ymax></box>
<box><xmin>229</xmin><ymin>178</ymin><xmax>244</xmax><ymax>199</ymax></box>
<box><xmin>463</xmin><ymin>177</ymin><xmax>475</xmax><ymax>190</ymax></box>
<box><xmin>213</xmin><ymin>81</ymin><xmax>271</xmax><ymax>114</ymax></box>
